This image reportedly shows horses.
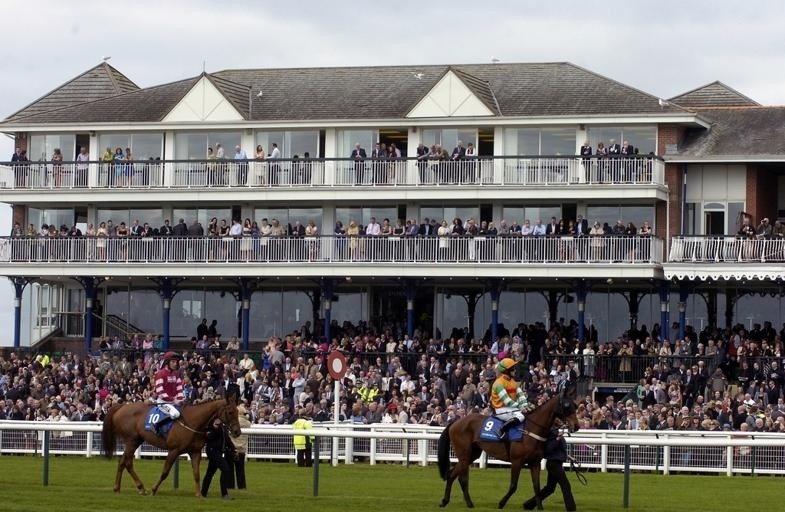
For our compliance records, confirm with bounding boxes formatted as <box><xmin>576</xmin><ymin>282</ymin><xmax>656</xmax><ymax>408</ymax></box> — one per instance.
<box><xmin>99</xmin><ymin>386</ymin><xmax>242</xmax><ymax>499</ymax></box>
<box><xmin>435</xmin><ymin>384</ymin><xmax>582</xmax><ymax>510</ymax></box>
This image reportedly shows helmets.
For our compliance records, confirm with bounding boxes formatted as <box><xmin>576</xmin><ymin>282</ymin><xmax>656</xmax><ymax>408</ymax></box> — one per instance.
<box><xmin>164</xmin><ymin>351</ymin><xmax>180</xmax><ymax>360</ymax></box>
<box><xmin>497</xmin><ymin>358</ymin><xmax>519</xmax><ymax>372</ymax></box>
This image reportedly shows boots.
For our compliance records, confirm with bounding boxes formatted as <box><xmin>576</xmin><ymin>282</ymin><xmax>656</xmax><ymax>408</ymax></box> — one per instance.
<box><xmin>152</xmin><ymin>416</ymin><xmax>171</xmax><ymax>435</ymax></box>
<box><xmin>495</xmin><ymin>417</ymin><xmax>520</xmax><ymax>437</ymax></box>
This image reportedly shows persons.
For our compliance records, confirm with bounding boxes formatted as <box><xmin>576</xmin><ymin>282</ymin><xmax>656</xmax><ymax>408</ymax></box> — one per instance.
<box><xmin>0</xmin><ymin>318</ymin><xmax>784</xmax><ymax>510</ymax></box>
<box><xmin>3</xmin><ymin>140</ymin><xmax>785</xmax><ymax>264</ymax></box>
